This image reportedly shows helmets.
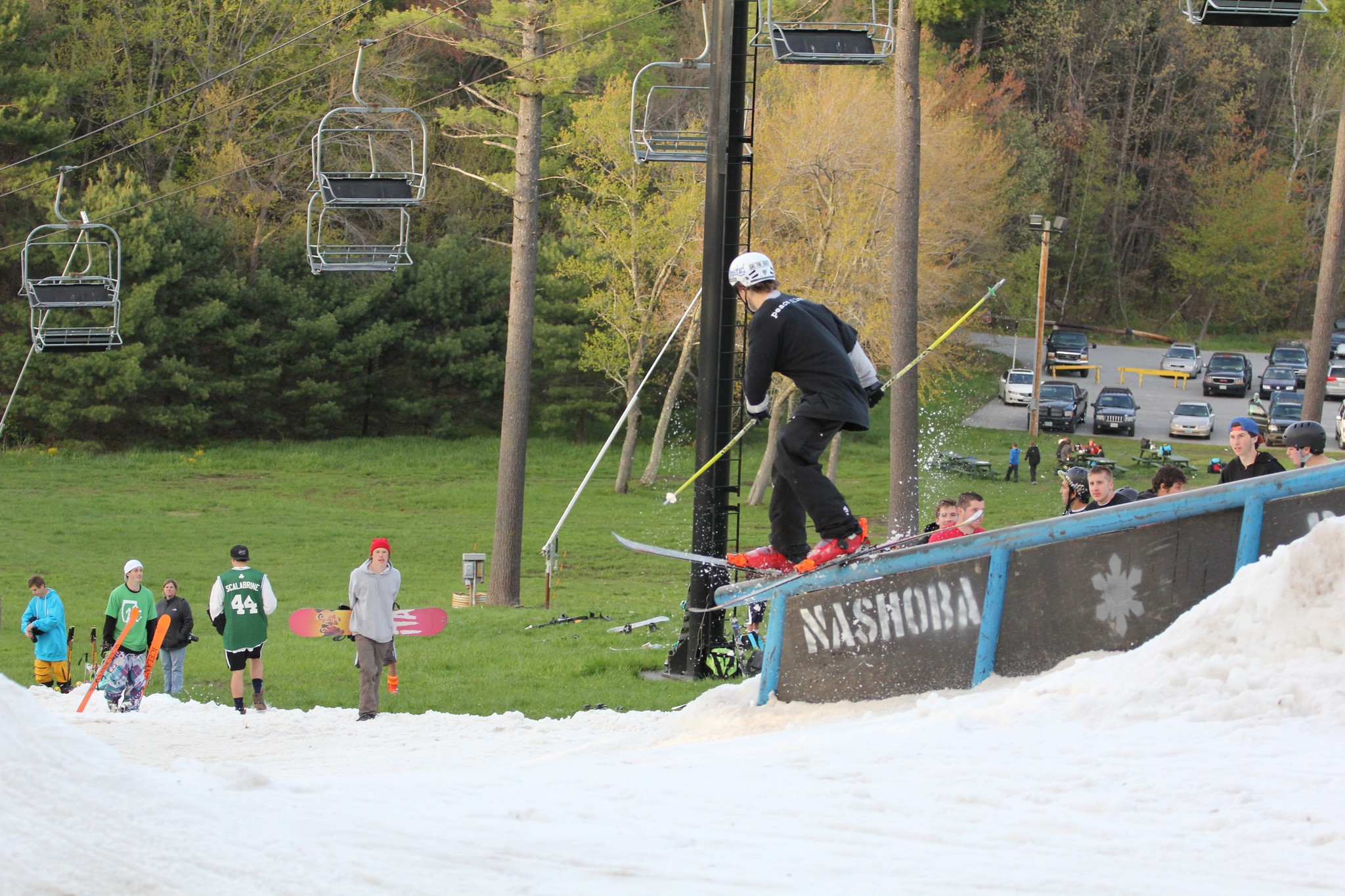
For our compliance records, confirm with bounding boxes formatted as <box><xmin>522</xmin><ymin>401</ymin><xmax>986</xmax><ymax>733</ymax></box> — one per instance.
<box><xmin>1064</xmin><ymin>466</ymin><xmax>1091</xmax><ymax>500</ymax></box>
<box><xmin>1282</xmin><ymin>420</ymin><xmax>1327</xmax><ymax>453</ymax></box>
<box><xmin>1113</xmin><ymin>487</ymin><xmax>1139</xmax><ymax>500</ymax></box>
<box><xmin>728</xmin><ymin>252</ymin><xmax>776</xmax><ymax>287</ymax></box>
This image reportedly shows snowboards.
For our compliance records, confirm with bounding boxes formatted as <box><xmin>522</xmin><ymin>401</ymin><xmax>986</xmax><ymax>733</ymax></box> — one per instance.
<box><xmin>288</xmin><ymin>608</ymin><xmax>449</xmax><ymax>639</ymax></box>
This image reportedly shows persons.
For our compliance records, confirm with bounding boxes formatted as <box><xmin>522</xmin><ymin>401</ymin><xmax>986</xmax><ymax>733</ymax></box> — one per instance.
<box><xmin>1249</xmin><ymin>392</ymin><xmax>1262</xmax><ymax>415</ymax></box>
<box><xmin>927</xmin><ymin>492</ymin><xmax>987</xmax><ymax>543</ymax></box>
<box><xmin>1134</xmin><ymin>464</ymin><xmax>1186</xmax><ymax>501</ymax></box>
<box><xmin>349</xmin><ymin>538</ymin><xmax>399</xmax><ymax>721</ymax></box>
<box><xmin>1004</xmin><ymin>443</ymin><xmax>1021</xmax><ymax>483</ymax></box>
<box><xmin>154</xmin><ymin>578</ymin><xmax>194</xmax><ymax>694</ymax></box>
<box><xmin>915</xmin><ymin>498</ymin><xmax>959</xmax><ymax>545</ymax></box>
<box><xmin>1282</xmin><ymin>420</ymin><xmax>1337</xmax><ymax>468</ymax></box>
<box><xmin>889</xmin><ymin>545</ymin><xmax>900</xmax><ymax>551</ymax></box>
<box><xmin>1025</xmin><ymin>440</ymin><xmax>1041</xmax><ymax>485</ymax></box>
<box><xmin>727</xmin><ymin>252</ymin><xmax>883</xmax><ymax>576</ymax></box>
<box><xmin>1218</xmin><ymin>417</ymin><xmax>1285</xmax><ymax>485</ymax></box>
<box><xmin>208</xmin><ymin>544</ymin><xmax>277</xmax><ymax>714</ymax></box>
<box><xmin>744</xmin><ymin>571</ymin><xmax>767</xmax><ymax>634</ymax></box>
<box><xmin>101</xmin><ymin>560</ymin><xmax>159</xmax><ymax>712</ymax></box>
<box><xmin>1055</xmin><ymin>436</ymin><xmax>1104</xmax><ymax>468</ymax></box>
<box><xmin>1057</xmin><ymin>465</ymin><xmax>1132</xmax><ymax>516</ymax></box>
<box><xmin>21</xmin><ymin>576</ymin><xmax>72</xmax><ymax>693</ymax></box>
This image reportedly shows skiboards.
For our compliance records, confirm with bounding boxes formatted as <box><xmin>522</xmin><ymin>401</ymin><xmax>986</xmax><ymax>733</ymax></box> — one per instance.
<box><xmin>611</xmin><ymin>509</ymin><xmax>984</xmax><ymax>611</ymax></box>
<box><xmin>729</xmin><ymin>615</ymin><xmax>750</xmax><ymax>678</ymax></box>
<box><xmin>606</xmin><ymin>615</ymin><xmax>670</xmax><ymax>651</ymax></box>
<box><xmin>523</xmin><ymin>615</ymin><xmax>589</xmax><ymax>645</ymax></box>
<box><xmin>74</xmin><ymin>607</ymin><xmax>171</xmax><ymax>712</ymax></box>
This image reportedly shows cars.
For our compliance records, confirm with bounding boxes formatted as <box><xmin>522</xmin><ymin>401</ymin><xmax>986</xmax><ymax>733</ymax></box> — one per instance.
<box><xmin>1168</xmin><ymin>401</ymin><xmax>1215</xmax><ymax>440</ymax></box>
<box><xmin>1258</xmin><ymin>365</ymin><xmax>1300</xmax><ymax>400</ymax></box>
<box><xmin>999</xmin><ymin>368</ymin><xmax>1034</xmax><ymax>406</ymax></box>
<box><xmin>1159</xmin><ymin>340</ymin><xmax>1202</xmax><ymax>380</ymax></box>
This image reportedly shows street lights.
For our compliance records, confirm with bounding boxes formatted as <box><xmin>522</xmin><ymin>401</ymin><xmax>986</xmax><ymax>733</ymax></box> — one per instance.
<box><xmin>1027</xmin><ymin>214</ymin><xmax>1069</xmax><ymax>447</ymax></box>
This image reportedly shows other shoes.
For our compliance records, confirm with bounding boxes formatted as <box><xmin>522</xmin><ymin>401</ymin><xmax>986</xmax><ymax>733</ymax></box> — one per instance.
<box><xmin>358</xmin><ymin>714</ymin><xmax>376</xmax><ymax>722</ymax></box>
<box><xmin>743</xmin><ymin>544</ymin><xmax>797</xmax><ymax>572</ymax></box>
<box><xmin>808</xmin><ymin>528</ymin><xmax>864</xmax><ymax>564</ymax></box>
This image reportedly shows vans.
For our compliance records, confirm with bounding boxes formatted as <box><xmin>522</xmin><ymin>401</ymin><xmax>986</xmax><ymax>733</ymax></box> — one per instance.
<box><xmin>1335</xmin><ymin>399</ymin><xmax>1345</xmax><ymax>450</ymax></box>
<box><xmin>1325</xmin><ymin>359</ymin><xmax>1345</xmax><ymax>399</ymax></box>
<box><xmin>1264</xmin><ymin>341</ymin><xmax>1310</xmax><ymax>388</ymax></box>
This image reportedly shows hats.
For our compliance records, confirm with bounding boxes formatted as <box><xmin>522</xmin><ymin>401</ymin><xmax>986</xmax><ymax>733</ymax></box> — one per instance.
<box><xmin>230</xmin><ymin>545</ymin><xmax>250</xmax><ymax>561</ymax></box>
<box><xmin>1058</xmin><ymin>470</ymin><xmax>1067</xmax><ymax>482</ymax></box>
<box><xmin>370</xmin><ymin>538</ymin><xmax>390</xmax><ymax>553</ymax></box>
<box><xmin>124</xmin><ymin>559</ymin><xmax>143</xmax><ymax>582</ymax></box>
<box><xmin>1228</xmin><ymin>418</ymin><xmax>1265</xmax><ymax>444</ymax></box>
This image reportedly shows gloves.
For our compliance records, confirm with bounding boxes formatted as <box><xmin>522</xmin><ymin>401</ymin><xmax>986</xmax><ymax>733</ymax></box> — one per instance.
<box><xmin>747</xmin><ymin>409</ymin><xmax>769</xmax><ymax>425</ymax></box>
<box><xmin>864</xmin><ymin>382</ymin><xmax>884</xmax><ymax>410</ymax></box>
<box><xmin>101</xmin><ymin>641</ymin><xmax>110</xmax><ymax>659</ymax></box>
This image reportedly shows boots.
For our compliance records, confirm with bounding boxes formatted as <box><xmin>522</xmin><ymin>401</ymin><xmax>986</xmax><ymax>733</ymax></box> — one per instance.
<box><xmin>386</xmin><ymin>675</ymin><xmax>399</xmax><ymax>695</ymax></box>
<box><xmin>251</xmin><ymin>691</ymin><xmax>266</xmax><ymax>710</ymax></box>
<box><xmin>236</xmin><ymin>703</ymin><xmax>245</xmax><ymax>715</ymax></box>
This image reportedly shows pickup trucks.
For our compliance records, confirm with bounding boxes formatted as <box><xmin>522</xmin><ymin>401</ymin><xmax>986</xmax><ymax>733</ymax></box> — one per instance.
<box><xmin>1199</xmin><ymin>352</ymin><xmax>1251</xmax><ymax>398</ymax></box>
<box><xmin>1027</xmin><ymin>381</ymin><xmax>1089</xmax><ymax>433</ymax></box>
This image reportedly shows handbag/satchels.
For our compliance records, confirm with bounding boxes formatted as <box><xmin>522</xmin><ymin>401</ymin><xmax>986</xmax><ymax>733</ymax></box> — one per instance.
<box><xmin>1208</xmin><ymin>458</ymin><xmax>1222</xmax><ymax>474</ymax></box>
<box><xmin>1159</xmin><ymin>445</ymin><xmax>1172</xmax><ymax>456</ymax></box>
<box><xmin>1140</xmin><ymin>438</ymin><xmax>1151</xmax><ymax>450</ymax></box>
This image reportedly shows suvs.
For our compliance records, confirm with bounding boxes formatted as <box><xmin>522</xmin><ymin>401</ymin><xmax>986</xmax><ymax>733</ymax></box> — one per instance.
<box><xmin>1044</xmin><ymin>330</ymin><xmax>1096</xmax><ymax>378</ymax></box>
<box><xmin>1329</xmin><ymin>319</ymin><xmax>1345</xmax><ymax>357</ymax></box>
<box><xmin>1091</xmin><ymin>386</ymin><xmax>1141</xmax><ymax>437</ymax></box>
<box><xmin>1247</xmin><ymin>391</ymin><xmax>1304</xmax><ymax>447</ymax></box>
